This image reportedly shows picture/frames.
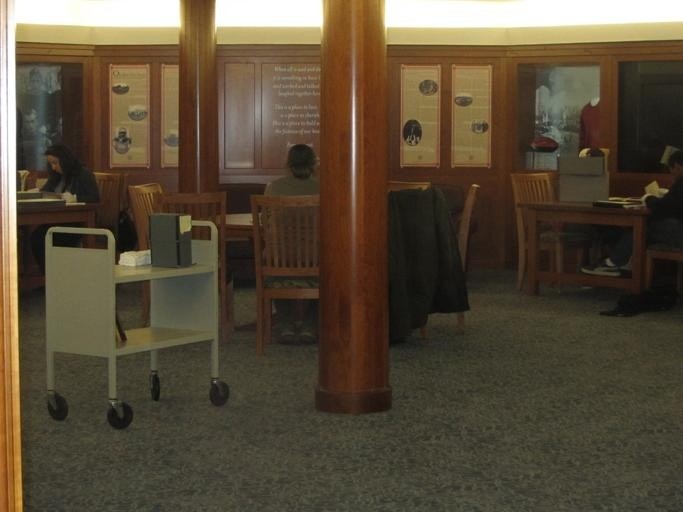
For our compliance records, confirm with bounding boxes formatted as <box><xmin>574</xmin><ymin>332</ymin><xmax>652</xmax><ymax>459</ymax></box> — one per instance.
<box><xmin>399</xmin><ymin>64</ymin><xmax>442</xmax><ymax>169</ymax></box>
<box><xmin>451</xmin><ymin>63</ymin><xmax>492</xmax><ymax>170</ymax></box>
<box><xmin>109</xmin><ymin>63</ymin><xmax>151</xmax><ymax>169</ymax></box>
<box><xmin>161</xmin><ymin>64</ymin><xmax>179</xmax><ymax>169</ymax></box>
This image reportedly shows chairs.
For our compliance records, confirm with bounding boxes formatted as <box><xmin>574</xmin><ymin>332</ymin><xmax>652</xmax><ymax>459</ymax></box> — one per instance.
<box><xmin>18</xmin><ymin>170</ymin><xmax>126</xmax><ymax>292</ymax></box>
<box><xmin>646</xmin><ymin>243</ymin><xmax>683</xmax><ymax>294</ymax></box>
<box><xmin>250</xmin><ymin>192</ymin><xmax>320</xmax><ymax>354</ymax></box>
<box><xmin>511</xmin><ymin>172</ymin><xmax>602</xmax><ymax>293</ymax></box>
<box><xmin>422</xmin><ymin>183</ymin><xmax>480</xmax><ymax>337</ymax></box>
<box><xmin>155</xmin><ymin>190</ymin><xmax>235</xmax><ymax>342</ymax></box>
<box><xmin>129</xmin><ymin>183</ymin><xmax>165</xmax><ymax>325</ymax></box>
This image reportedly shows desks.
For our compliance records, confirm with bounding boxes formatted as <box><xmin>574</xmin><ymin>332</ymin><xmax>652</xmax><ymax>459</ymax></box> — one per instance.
<box><xmin>518</xmin><ymin>198</ymin><xmax>651</xmax><ymax>300</ymax></box>
<box><xmin>207</xmin><ymin>214</ymin><xmax>270</xmax><ymax>242</ymax></box>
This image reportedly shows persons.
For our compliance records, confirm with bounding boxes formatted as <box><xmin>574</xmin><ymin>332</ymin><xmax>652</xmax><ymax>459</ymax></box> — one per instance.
<box><xmin>30</xmin><ymin>143</ymin><xmax>100</xmax><ymax>296</ymax></box>
<box><xmin>115</xmin><ymin>128</ymin><xmax>131</xmax><ymax>144</ymax></box>
<box><xmin>261</xmin><ymin>144</ymin><xmax>321</xmax><ymax>320</ymax></box>
<box><xmin>580</xmin><ymin>150</ymin><xmax>683</xmax><ymax>276</ymax></box>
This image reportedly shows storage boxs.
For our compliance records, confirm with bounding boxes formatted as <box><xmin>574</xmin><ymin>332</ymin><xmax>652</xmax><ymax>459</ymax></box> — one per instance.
<box><xmin>559</xmin><ymin>143</ymin><xmax>609</xmax><ymax>176</ymax></box>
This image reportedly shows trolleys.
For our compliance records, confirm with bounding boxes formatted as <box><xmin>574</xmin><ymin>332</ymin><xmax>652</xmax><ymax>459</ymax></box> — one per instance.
<box><xmin>44</xmin><ymin>221</ymin><xmax>229</xmax><ymax>428</ymax></box>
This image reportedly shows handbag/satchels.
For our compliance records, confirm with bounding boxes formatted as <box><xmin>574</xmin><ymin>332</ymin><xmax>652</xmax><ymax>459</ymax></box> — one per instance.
<box><xmin>118</xmin><ymin>213</ymin><xmax>136</xmax><ymax>251</ymax></box>
